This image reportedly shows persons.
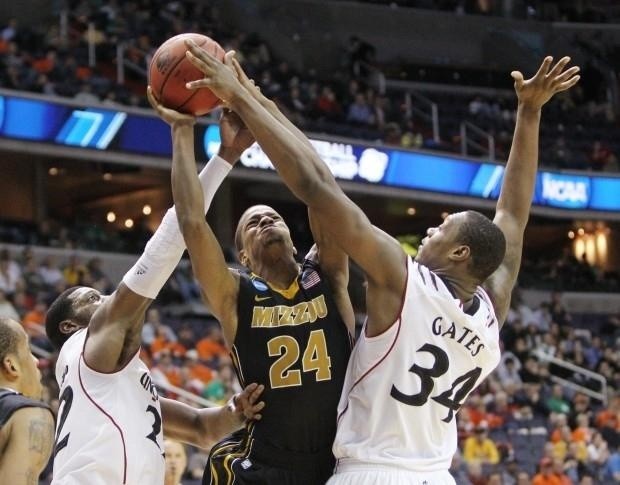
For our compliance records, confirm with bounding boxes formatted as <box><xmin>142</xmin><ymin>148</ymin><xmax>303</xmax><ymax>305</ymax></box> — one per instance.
<box><xmin>147</xmin><ymin>57</ymin><xmax>356</xmax><ymax>485</ymax></box>
<box><xmin>181</xmin><ymin>37</ymin><xmax>580</xmax><ymax>484</ymax></box>
<box><xmin>2</xmin><ymin>80</ymin><xmax>266</xmax><ymax>485</ymax></box>
<box><xmin>2</xmin><ymin>2</ymin><xmax>619</xmax><ymax>171</ymax></box>
<box><xmin>449</xmin><ymin>247</ymin><xmax>618</xmax><ymax>484</ymax></box>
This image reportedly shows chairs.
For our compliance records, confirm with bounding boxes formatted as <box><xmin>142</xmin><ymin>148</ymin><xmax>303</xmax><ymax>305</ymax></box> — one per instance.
<box><xmin>486</xmin><ymin>420</ymin><xmax>556</xmax><ymax>477</ymax></box>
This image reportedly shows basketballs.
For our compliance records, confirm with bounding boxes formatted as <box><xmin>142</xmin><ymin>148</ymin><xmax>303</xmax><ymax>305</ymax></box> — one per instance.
<box><xmin>150</xmin><ymin>33</ymin><xmax>229</xmax><ymax>115</ymax></box>
